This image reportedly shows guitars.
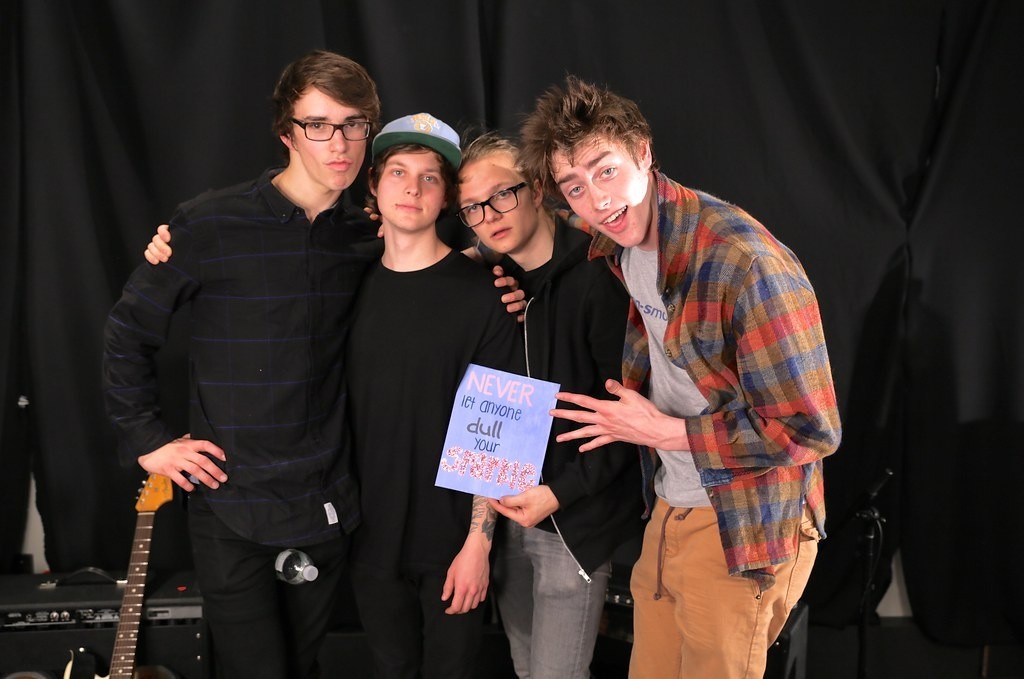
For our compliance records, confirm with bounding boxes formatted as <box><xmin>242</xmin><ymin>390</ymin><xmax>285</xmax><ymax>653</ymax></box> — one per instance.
<box><xmin>108</xmin><ymin>473</ymin><xmax>175</xmax><ymax>679</ymax></box>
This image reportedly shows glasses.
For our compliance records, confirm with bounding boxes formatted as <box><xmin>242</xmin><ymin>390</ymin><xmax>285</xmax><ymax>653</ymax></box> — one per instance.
<box><xmin>452</xmin><ymin>181</ymin><xmax>527</xmax><ymax>228</ymax></box>
<box><xmin>289</xmin><ymin>113</ymin><xmax>373</xmax><ymax>141</ymax></box>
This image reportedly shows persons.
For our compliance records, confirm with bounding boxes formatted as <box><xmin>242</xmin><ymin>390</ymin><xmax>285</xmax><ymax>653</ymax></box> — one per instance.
<box><xmin>363</xmin><ymin>132</ymin><xmax>649</xmax><ymax>679</ymax></box>
<box><xmin>103</xmin><ymin>51</ymin><xmax>527</xmax><ymax>679</ymax></box>
<box><xmin>144</xmin><ymin>113</ymin><xmax>497</xmax><ymax>679</ymax></box>
<box><xmin>460</xmin><ymin>77</ymin><xmax>842</xmax><ymax>679</ymax></box>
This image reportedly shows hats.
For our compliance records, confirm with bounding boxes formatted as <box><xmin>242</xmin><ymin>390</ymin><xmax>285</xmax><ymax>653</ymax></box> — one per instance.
<box><xmin>370</xmin><ymin>113</ymin><xmax>463</xmax><ymax>172</ymax></box>
